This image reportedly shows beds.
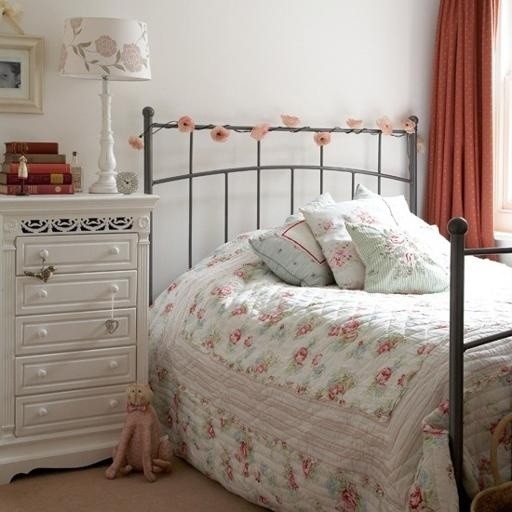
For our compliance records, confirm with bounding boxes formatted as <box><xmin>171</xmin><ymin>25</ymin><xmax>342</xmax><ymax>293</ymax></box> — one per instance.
<box><xmin>141</xmin><ymin>106</ymin><xmax>512</xmax><ymax>512</ymax></box>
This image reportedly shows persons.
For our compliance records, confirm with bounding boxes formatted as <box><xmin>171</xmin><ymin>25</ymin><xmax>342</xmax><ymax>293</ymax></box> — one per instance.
<box><xmin>1</xmin><ymin>61</ymin><xmax>21</xmax><ymax>87</ymax></box>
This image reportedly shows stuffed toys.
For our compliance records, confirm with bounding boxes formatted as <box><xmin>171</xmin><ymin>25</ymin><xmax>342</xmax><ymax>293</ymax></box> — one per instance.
<box><xmin>106</xmin><ymin>383</ymin><xmax>174</xmax><ymax>481</ymax></box>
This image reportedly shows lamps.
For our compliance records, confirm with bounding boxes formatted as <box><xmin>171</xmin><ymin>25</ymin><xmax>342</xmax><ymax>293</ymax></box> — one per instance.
<box><xmin>57</xmin><ymin>16</ymin><xmax>152</xmax><ymax>194</ymax></box>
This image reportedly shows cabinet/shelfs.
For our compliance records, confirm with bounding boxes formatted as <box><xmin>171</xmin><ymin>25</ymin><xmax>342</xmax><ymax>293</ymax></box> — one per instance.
<box><xmin>1</xmin><ymin>193</ymin><xmax>160</xmax><ymax>488</ymax></box>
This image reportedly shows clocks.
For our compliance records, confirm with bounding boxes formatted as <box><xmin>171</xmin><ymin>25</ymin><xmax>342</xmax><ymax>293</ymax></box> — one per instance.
<box><xmin>116</xmin><ymin>172</ymin><xmax>138</xmax><ymax>194</ymax></box>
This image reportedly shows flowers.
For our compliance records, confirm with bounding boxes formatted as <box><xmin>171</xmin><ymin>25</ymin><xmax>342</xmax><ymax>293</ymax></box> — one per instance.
<box><xmin>128</xmin><ymin>114</ymin><xmax>426</xmax><ymax>155</ymax></box>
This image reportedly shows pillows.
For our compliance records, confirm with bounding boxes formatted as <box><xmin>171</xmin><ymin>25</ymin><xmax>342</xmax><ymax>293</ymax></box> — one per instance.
<box><xmin>248</xmin><ymin>191</ymin><xmax>338</xmax><ymax>287</ymax></box>
<box><xmin>352</xmin><ymin>184</ymin><xmax>440</xmax><ymax>236</ymax></box>
<box><xmin>341</xmin><ymin>212</ymin><xmax>450</xmax><ymax>294</ymax></box>
<box><xmin>298</xmin><ymin>194</ymin><xmax>411</xmax><ymax>290</ymax></box>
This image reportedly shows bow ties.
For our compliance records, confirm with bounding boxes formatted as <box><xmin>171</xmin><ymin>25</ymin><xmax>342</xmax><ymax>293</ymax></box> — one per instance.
<box><xmin>127</xmin><ymin>404</ymin><xmax>146</xmax><ymax>413</ymax></box>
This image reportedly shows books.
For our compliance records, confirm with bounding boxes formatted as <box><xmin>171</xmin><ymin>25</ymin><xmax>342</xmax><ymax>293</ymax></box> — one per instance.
<box><xmin>1</xmin><ymin>142</ymin><xmax>74</xmax><ymax>194</ymax></box>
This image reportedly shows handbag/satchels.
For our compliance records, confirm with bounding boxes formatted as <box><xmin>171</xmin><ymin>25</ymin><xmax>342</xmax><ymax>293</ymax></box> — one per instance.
<box><xmin>469</xmin><ymin>411</ymin><xmax>512</xmax><ymax>512</ymax></box>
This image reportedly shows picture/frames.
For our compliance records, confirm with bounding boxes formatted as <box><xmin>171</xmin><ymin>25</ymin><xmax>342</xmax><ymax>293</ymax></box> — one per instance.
<box><xmin>0</xmin><ymin>34</ymin><xmax>46</xmax><ymax>114</ymax></box>
